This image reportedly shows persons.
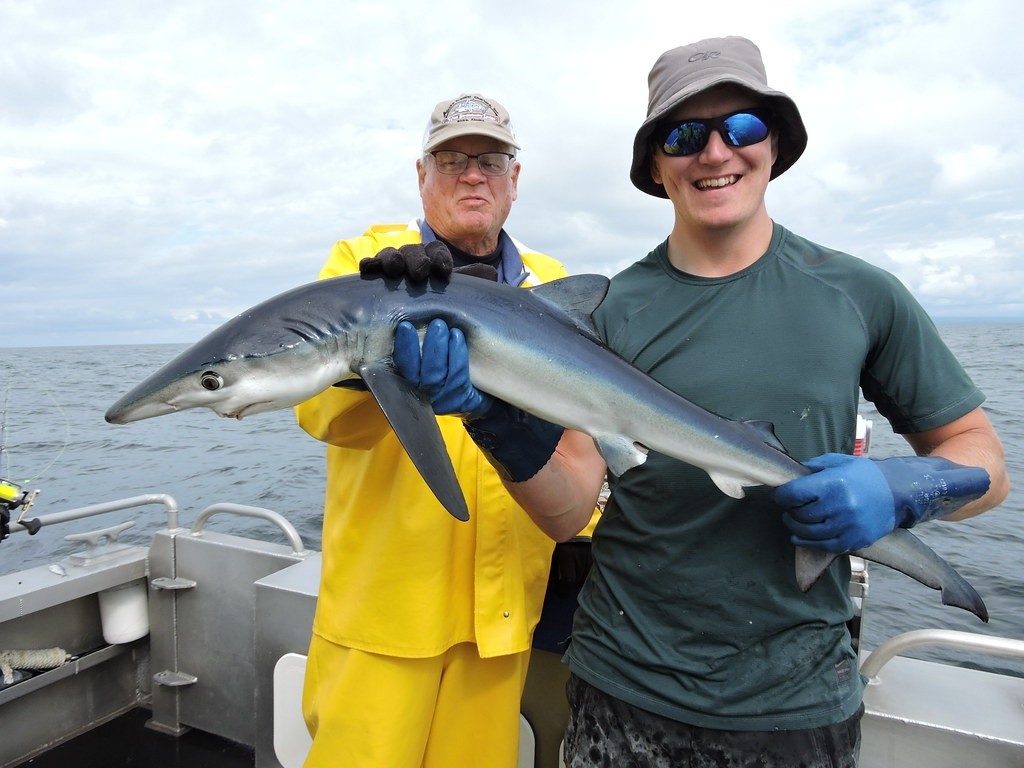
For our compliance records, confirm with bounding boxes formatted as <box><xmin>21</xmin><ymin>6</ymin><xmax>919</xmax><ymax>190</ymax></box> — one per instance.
<box><xmin>294</xmin><ymin>96</ymin><xmax>573</xmax><ymax>768</ymax></box>
<box><xmin>394</xmin><ymin>35</ymin><xmax>1009</xmax><ymax>768</ymax></box>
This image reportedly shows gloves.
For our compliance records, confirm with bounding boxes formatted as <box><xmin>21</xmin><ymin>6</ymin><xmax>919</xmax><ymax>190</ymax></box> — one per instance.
<box><xmin>393</xmin><ymin>322</ymin><xmax>564</xmax><ymax>482</ymax></box>
<box><xmin>777</xmin><ymin>455</ymin><xmax>988</xmax><ymax>551</ymax></box>
<box><xmin>359</xmin><ymin>241</ymin><xmax>498</xmax><ymax>285</ymax></box>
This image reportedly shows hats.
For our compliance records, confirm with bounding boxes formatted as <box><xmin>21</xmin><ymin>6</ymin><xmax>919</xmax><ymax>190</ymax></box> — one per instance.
<box><xmin>422</xmin><ymin>93</ymin><xmax>522</xmax><ymax>154</ymax></box>
<box><xmin>630</xmin><ymin>35</ymin><xmax>808</xmax><ymax>200</ymax></box>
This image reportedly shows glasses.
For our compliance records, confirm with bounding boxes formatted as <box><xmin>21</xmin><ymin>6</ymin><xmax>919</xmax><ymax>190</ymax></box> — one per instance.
<box><xmin>657</xmin><ymin>105</ymin><xmax>775</xmax><ymax>157</ymax></box>
<box><xmin>429</xmin><ymin>150</ymin><xmax>515</xmax><ymax>176</ymax></box>
